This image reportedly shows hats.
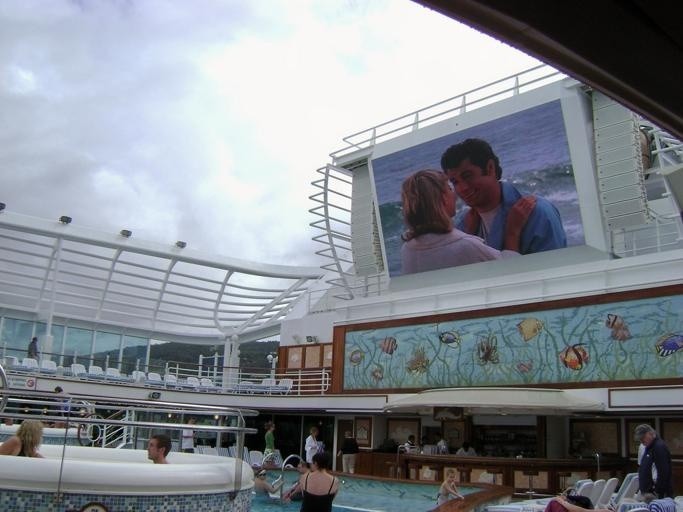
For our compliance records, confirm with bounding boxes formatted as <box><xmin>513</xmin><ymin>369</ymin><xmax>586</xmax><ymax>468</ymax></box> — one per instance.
<box><xmin>634</xmin><ymin>424</ymin><xmax>650</xmax><ymax>441</ymax></box>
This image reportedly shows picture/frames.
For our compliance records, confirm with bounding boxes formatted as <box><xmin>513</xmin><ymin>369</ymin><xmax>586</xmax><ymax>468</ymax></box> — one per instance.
<box><xmin>659</xmin><ymin>416</ymin><xmax>683</xmax><ymax>459</ymax></box>
<box><xmin>567</xmin><ymin>417</ymin><xmax>622</xmax><ymax>462</ymax></box>
<box><xmin>439</xmin><ymin>420</ymin><xmax>465</xmax><ymax>452</ymax></box>
<box><xmin>624</xmin><ymin>418</ymin><xmax>655</xmax><ymax>460</ymax></box>
<box><xmin>386</xmin><ymin>417</ymin><xmax>421</xmax><ymax>445</ymax></box>
<box><xmin>353</xmin><ymin>415</ymin><xmax>372</xmax><ymax>448</ymax></box>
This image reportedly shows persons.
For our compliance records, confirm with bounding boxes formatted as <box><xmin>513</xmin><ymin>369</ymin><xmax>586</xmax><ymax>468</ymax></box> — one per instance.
<box><xmin>633</xmin><ymin>423</ymin><xmax>672</xmax><ymax>498</ymax></box>
<box><xmin>541</xmin><ymin>492</ymin><xmax>658</xmax><ymax>512</ymax></box>
<box><xmin>3</xmin><ymin>415</ymin><xmax>14</xmax><ymax>425</ymax></box>
<box><xmin>26</xmin><ymin>336</ymin><xmax>40</xmax><ymax>362</ymax></box>
<box><xmin>0</xmin><ymin>418</ymin><xmax>45</xmax><ymax>458</ymax></box>
<box><xmin>438</xmin><ymin>136</ymin><xmax>567</xmax><ymax>253</ymax></box>
<box><xmin>298</xmin><ymin>453</ymin><xmax>339</xmax><ymax>511</ymax></box>
<box><xmin>53</xmin><ymin>386</ymin><xmax>72</xmax><ymax>428</ymax></box>
<box><xmin>336</xmin><ymin>429</ymin><xmax>359</xmax><ymax>474</ymax></box>
<box><xmin>146</xmin><ymin>433</ymin><xmax>171</xmax><ymax>463</ymax></box>
<box><xmin>403</xmin><ymin>429</ymin><xmax>511</xmax><ymax>458</ymax></box>
<box><xmin>263</xmin><ymin>420</ymin><xmax>276</xmax><ymax>467</ymax></box>
<box><xmin>437</xmin><ymin>468</ymin><xmax>464</xmax><ymax>505</ymax></box>
<box><xmin>304</xmin><ymin>425</ymin><xmax>323</xmax><ymax>469</ymax></box>
<box><xmin>252</xmin><ymin>467</ymin><xmax>286</xmax><ymax>497</ymax></box>
<box><xmin>286</xmin><ymin>461</ymin><xmax>311</xmax><ymax>501</ymax></box>
<box><xmin>182</xmin><ymin>415</ymin><xmax>197</xmax><ymax>453</ymax></box>
<box><xmin>397</xmin><ymin>168</ymin><xmax>537</xmax><ymax>274</ymax></box>
<box><xmin>636</xmin><ymin>442</ymin><xmax>658</xmax><ymax>490</ymax></box>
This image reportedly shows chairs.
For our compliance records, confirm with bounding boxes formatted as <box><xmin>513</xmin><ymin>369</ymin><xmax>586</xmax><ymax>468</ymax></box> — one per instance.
<box><xmin>483</xmin><ymin>472</ymin><xmax>682</xmax><ymax>512</ymax></box>
<box><xmin>0</xmin><ymin>355</ymin><xmax>295</xmax><ymax>396</ymax></box>
